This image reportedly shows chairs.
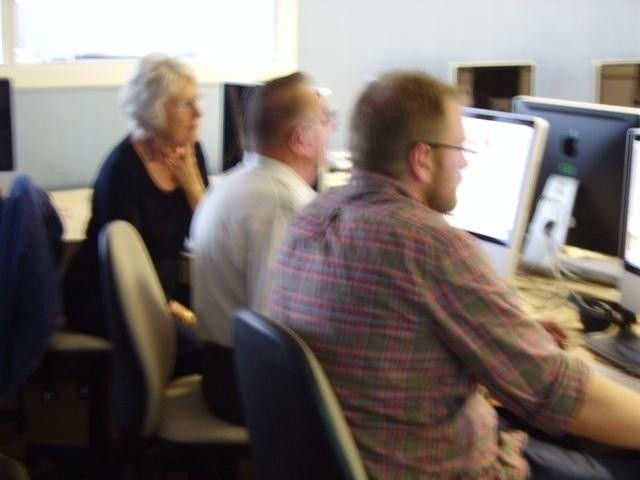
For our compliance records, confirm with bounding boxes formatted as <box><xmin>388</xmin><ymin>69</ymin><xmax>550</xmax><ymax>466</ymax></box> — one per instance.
<box><xmin>231</xmin><ymin>308</ymin><xmax>370</xmax><ymax>480</ymax></box>
<box><xmin>97</xmin><ymin>218</ymin><xmax>251</xmax><ymax>479</ymax></box>
<box><xmin>1</xmin><ymin>175</ymin><xmax>113</xmax><ymax>479</ymax></box>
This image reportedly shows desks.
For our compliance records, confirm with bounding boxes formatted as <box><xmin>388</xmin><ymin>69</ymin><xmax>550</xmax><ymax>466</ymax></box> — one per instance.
<box><xmin>478</xmin><ymin>245</ymin><xmax>640</xmax><ymax>480</ymax></box>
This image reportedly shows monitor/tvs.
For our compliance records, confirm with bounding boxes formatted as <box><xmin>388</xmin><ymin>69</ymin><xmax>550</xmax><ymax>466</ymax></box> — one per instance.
<box><xmin>444</xmin><ymin>106</ymin><xmax>550</xmax><ymax>279</ymax></box>
<box><xmin>511</xmin><ymin>95</ymin><xmax>640</xmax><ymax>279</ymax></box>
<box><xmin>583</xmin><ymin>127</ymin><xmax>640</xmax><ymax>377</ymax></box>
<box><xmin>216</xmin><ymin>81</ymin><xmax>331</xmax><ymax>195</ymax></box>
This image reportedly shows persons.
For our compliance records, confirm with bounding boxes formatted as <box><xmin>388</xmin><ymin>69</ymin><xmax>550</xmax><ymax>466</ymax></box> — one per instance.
<box><xmin>62</xmin><ymin>50</ymin><xmax>215</xmax><ymax>343</ymax></box>
<box><xmin>269</xmin><ymin>67</ymin><xmax>640</xmax><ymax>480</ymax></box>
<box><xmin>191</xmin><ymin>70</ymin><xmax>332</xmax><ymax>428</ymax></box>
